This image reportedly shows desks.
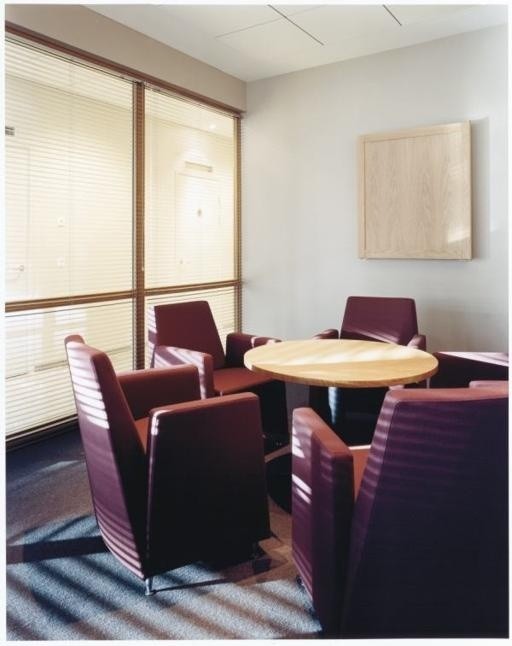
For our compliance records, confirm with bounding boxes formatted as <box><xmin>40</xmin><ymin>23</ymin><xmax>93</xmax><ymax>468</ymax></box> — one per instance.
<box><xmin>244</xmin><ymin>339</ymin><xmax>440</xmax><ymax>429</ymax></box>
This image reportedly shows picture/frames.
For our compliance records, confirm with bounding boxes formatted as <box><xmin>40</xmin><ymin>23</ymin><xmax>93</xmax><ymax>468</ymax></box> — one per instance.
<box><xmin>357</xmin><ymin>121</ymin><xmax>474</xmax><ymax>260</ymax></box>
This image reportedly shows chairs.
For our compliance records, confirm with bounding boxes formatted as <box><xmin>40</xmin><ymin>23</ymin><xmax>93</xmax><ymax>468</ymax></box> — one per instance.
<box><xmin>435</xmin><ymin>351</ymin><xmax>510</xmax><ymax>387</ymax></box>
<box><xmin>141</xmin><ymin>299</ymin><xmax>291</xmax><ymax>475</ymax></box>
<box><xmin>65</xmin><ymin>335</ymin><xmax>271</xmax><ymax>597</ymax></box>
<box><xmin>286</xmin><ymin>387</ymin><xmax>507</xmax><ymax>638</ymax></box>
<box><xmin>300</xmin><ymin>296</ymin><xmax>425</xmax><ymax>444</ymax></box>
